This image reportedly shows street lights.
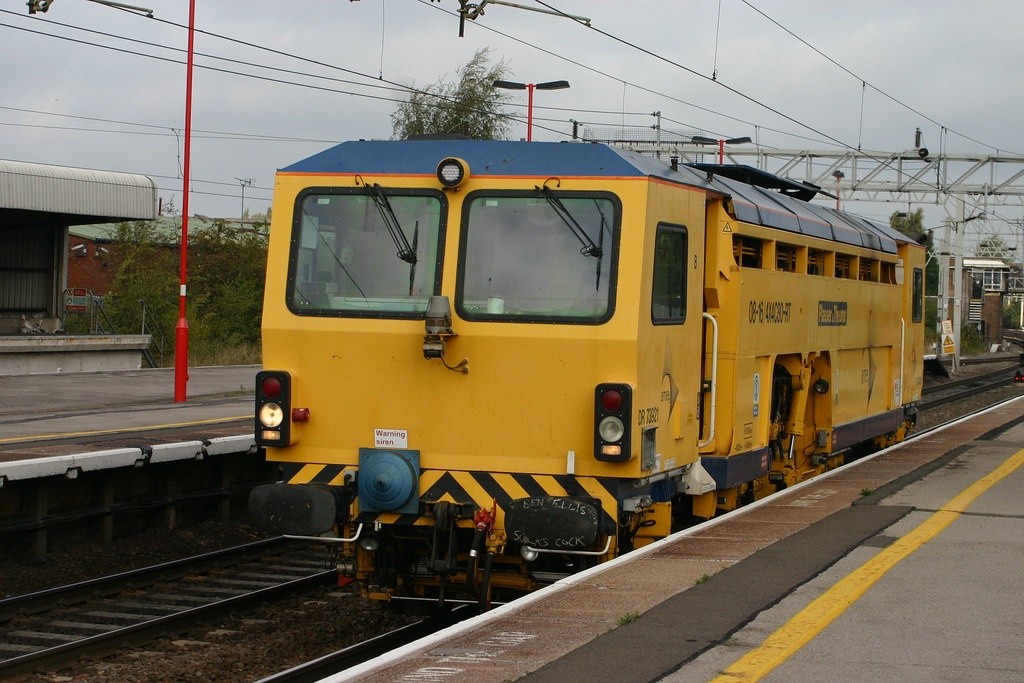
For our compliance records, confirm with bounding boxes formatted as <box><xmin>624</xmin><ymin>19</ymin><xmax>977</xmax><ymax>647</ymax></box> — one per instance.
<box><xmin>493</xmin><ymin>80</ymin><xmax>571</xmax><ymax>142</ymax></box>
<box><xmin>690</xmin><ymin>136</ymin><xmax>753</xmax><ymax>165</ymax></box>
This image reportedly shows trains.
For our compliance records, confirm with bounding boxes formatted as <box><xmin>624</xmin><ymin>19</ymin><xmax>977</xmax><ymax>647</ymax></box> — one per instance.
<box><xmin>245</xmin><ymin>140</ymin><xmax>929</xmax><ymax>619</ymax></box>
